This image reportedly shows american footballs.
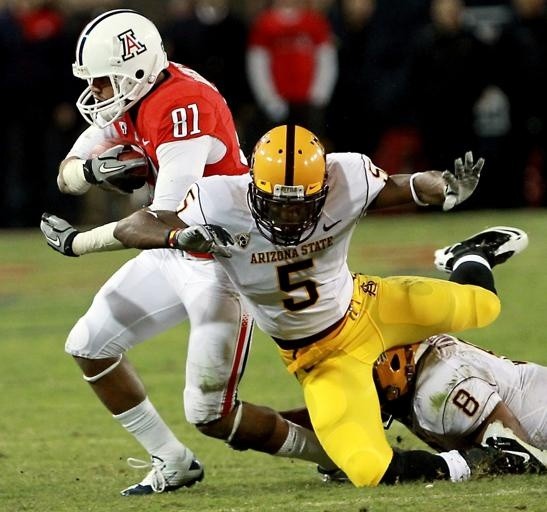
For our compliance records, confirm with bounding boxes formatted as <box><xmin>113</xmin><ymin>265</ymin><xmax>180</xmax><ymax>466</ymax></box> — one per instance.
<box><xmin>88</xmin><ymin>139</ymin><xmax>149</xmax><ymax>177</ymax></box>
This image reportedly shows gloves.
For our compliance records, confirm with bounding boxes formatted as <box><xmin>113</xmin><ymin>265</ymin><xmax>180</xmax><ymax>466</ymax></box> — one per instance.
<box><xmin>83</xmin><ymin>145</ymin><xmax>147</xmax><ymax>196</ymax></box>
<box><xmin>164</xmin><ymin>223</ymin><xmax>232</xmax><ymax>257</ymax></box>
<box><xmin>40</xmin><ymin>214</ymin><xmax>79</xmax><ymax>256</ymax></box>
<box><xmin>443</xmin><ymin>151</ymin><xmax>485</xmax><ymax>211</ymax></box>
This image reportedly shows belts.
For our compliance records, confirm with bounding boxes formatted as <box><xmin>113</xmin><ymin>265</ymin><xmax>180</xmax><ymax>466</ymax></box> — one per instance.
<box><xmin>288</xmin><ymin>280</ymin><xmax>362</xmax><ymax>375</ymax></box>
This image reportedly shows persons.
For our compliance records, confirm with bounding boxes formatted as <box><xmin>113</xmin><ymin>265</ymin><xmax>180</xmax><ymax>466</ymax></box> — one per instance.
<box><xmin>278</xmin><ymin>336</ymin><xmax>546</xmax><ymax>471</ymax></box>
<box><xmin>3</xmin><ymin>0</ymin><xmax>546</xmax><ymax>229</ymax></box>
<box><xmin>40</xmin><ymin>124</ymin><xmax>529</xmax><ymax>487</ymax></box>
<box><xmin>39</xmin><ymin>9</ymin><xmax>351</xmax><ymax>498</ymax></box>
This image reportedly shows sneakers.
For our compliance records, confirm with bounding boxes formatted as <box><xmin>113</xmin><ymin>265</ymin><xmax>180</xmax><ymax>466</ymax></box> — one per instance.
<box><xmin>481</xmin><ymin>419</ymin><xmax>546</xmax><ymax>473</ymax></box>
<box><xmin>121</xmin><ymin>448</ymin><xmax>203</xmax><ymax>495</ymax></box>
<box><xmin>433</xmin><ymin>225</ymin><xmax>530</xmax><ymax>275</ymax></box>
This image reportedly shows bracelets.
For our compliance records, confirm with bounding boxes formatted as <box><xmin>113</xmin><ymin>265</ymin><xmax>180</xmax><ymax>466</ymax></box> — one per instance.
<box><xmin>164</xmin><ymin>225</ymin><xmax>180</xmax><ymax>249</ymax></box>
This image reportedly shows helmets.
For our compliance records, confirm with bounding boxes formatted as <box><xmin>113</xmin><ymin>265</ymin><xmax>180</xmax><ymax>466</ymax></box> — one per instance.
<box><xmin>246</xmin><ymin>125</ymin><xmax>328</xmax><ymax>245</ymax></box>
<box><xmin>73</xmin><ymin>10</ymin><xmax>168</xmax><ymax>129</ymax></box>
<box><xmin>373</xmin><ymin>345</ymin><xmax>417</xmax><ymax>401</ymax></box>
